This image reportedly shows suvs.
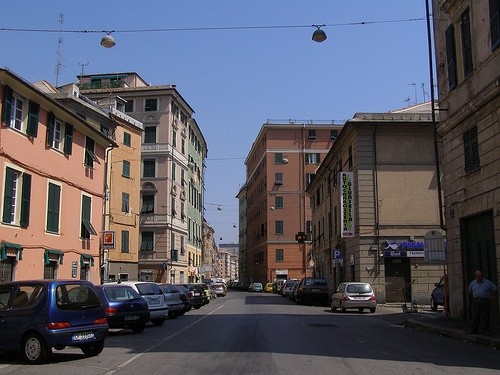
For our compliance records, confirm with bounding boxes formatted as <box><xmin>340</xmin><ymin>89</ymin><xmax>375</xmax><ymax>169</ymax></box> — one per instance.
<box><xmin>272</xmin><ymin>277</ymin><xmax>330</xmax><ymax>305</ymax></box>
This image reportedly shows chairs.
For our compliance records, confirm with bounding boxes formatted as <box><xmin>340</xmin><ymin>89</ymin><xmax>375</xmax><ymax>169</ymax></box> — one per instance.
<box><xmin>13</xmin><ymin>291</ymin><xmax>28</xmax><ymax>306</ymax></box>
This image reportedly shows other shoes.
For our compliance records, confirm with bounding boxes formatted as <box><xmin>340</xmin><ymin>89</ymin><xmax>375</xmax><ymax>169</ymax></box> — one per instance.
<box><xmin>466</xmin><ymin>329</ymin><xmax>473</xmax><ymax>334</ymax></box>
<box><xmin>484</xmin><ymin>331</ymin><xmax>492</xmax><ymax>336</ymax></box>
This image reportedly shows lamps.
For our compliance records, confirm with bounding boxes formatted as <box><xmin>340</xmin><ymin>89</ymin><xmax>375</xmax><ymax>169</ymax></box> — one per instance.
<box><xmin>282</xmin><ymin>154</ymin><xmax>288</xmax><ymax>165</ymax></box>
<box><xmin>100</xmin><ymin>30</ymin><xmax>116</xmax><ymax>48</ymax></box>
<box><xmin>312</xmin><ymin>24</ymin><xmax>327</xmax><ymax>43</ymax></box>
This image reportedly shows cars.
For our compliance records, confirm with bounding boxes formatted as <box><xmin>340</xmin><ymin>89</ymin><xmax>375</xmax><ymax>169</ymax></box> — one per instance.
<box><xmin>207</xmin><ymin>281</ymin><xmax>228</xmax><ymax>299</ymax></box>
<box><xmin>264</xmin><ymin>283</ymin><xmax>273</xmax><ymax>292</ymax></box>
<box><xmin>331</xmin><ymin>282</ymin><xmax>377</xmax><ymax>313</ymax></box>
<box><xmin>430</xmin><ymin>274</ymin><xmax>448</xmax><ymax>311</ymax></box>
<box><xmin>156</xmin><ymin>283</ymin><xmax>209</xmax><ymax>318</ymax></box>
<box><xmin>232</xmin><ymin>282</ymin><xmax>263</xmax><ymax>291</ymax></box>
<box><xmin>0</xmin><ymin>280</ymin><xmax>109</xmax><ymax>364</ymax></box>
<box><xmin>59</xmin><ymin>280</ymin><xmax>169</xmax><ymax>333</ymax></box>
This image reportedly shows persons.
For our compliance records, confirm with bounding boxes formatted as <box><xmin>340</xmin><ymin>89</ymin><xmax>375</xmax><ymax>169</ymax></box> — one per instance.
<box><xmin>465</xmin><ymin>269</ymin><xmax>498</xmax><ymax>335</ymax></box>
